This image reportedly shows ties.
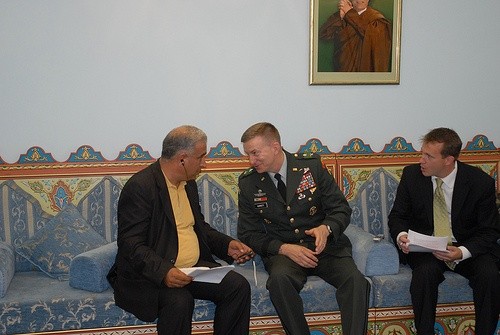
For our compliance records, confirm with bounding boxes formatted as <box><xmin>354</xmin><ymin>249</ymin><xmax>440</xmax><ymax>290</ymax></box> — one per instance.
<box><xmin>433</xmin><ymin>178</ymin><xmax>456</xmax><ymax>271</ymax></box>
<box><xmin>274</xmin><ymin>173</ymin><xmax>287</xmax><ymax>204</ymax></box>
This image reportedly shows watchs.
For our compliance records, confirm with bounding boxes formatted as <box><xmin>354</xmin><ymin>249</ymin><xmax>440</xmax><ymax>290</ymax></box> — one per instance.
<box><xmin>327</xmin><ymin>225</ymin><xmax>332</xmax><ymax>233</ymax></box>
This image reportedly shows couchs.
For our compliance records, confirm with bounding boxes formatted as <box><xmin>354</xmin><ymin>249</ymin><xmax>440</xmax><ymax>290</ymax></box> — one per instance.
<box><xmin>0</xmin><ymin>134</ymin><xmax>500</xmax><ymax>335</ymax></box>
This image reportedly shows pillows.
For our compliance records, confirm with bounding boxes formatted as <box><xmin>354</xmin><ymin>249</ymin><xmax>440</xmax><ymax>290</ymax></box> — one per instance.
<box><xmin>14</xmin><ymin>202</ymin><xmax>110</xmax><ymax>285</ymax></box>
<box><xmin>341</xmin><ymin>225</ymin><xmax>401</xmax><ymax>279</ymax></box>
<box><xmin>67</xmin><ymin>242</ymin><xmax>118</xmax><ymax>294</ymax></box>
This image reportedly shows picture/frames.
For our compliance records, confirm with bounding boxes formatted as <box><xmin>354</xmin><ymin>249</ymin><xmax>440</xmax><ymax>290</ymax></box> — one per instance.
<box><xmin>309</xmin><ymin>0</ymin><xmax>404</xmax><ymax>89</ymax></box>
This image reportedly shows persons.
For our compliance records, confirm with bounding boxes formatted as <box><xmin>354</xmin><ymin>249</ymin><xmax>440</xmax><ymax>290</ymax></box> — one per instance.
<box><xmin>106</xmin><ymin>125</ymin><xmax>254</xmax><ymax>335</ymax></box>
<box><xmin>237</xmin><ymin>122</ymin><xmax>371</xmax><ymax>335</ymax></box>
<box><xmin>319</xmin><ymin>0</ymin><xmax>392</xmax><ymax>72</ymax></box>
<box><xmin>387</xmin><ymin>128</ymin><xmax>500</xmax><ymax>335</ymax></box>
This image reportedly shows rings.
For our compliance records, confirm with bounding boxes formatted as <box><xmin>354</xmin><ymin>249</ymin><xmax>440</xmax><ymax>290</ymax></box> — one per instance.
<box><xmin>399</xmin><ymin>242</ymin><xmax>404</xmax><ymax>246</ymax></box>
